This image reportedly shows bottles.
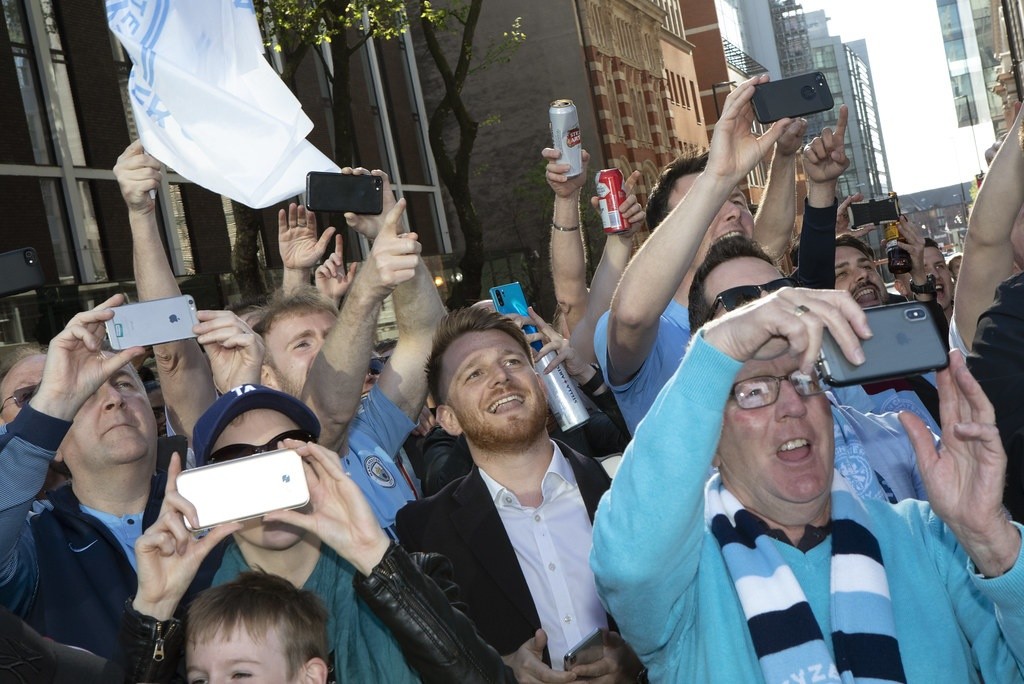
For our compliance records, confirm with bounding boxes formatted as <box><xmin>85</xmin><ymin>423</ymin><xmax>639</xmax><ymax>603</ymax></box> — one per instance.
<box><xmin>883</xmin><ymin>192</ymin><xmax>913</xmax><ymax>274</ymax></box>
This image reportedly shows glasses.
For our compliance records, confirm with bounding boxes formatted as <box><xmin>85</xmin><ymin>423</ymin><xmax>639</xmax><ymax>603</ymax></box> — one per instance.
<box><xmin>367</xmin><ymin>356</ymin><xmax>387</xmax><ymax>376</ymax></box>
<box><xmin>0</xmin><ymin>385</ymin><xmax>39</xmax><ymax>413</ymax></box>
<box><xmin>729</xmin><ymin>358</ymin><xmax>834</xmax><ymax>409</ymax></box>
<box><xmin>704</xmin><ymin>277</ymin><xmax>802</xmax><ymax>323</ymax></box>
<box><xmin>204</xmin><ymin>430</ymin><xmax>316</xmax><ymax>466</ymax></box>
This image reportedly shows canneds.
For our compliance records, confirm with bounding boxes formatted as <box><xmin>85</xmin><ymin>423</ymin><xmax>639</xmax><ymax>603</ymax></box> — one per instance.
<box><xmin>550</xmin><ymin>99</ymin><xmax>583</xmax><ymax>178</ymax></box>
<box><xmin>534</xmin><ymin>348</ymin><xmax>591</xmax><ymax>432</ymax></box>
<box><xmin>595</xmin><ymin>168</ymin><xmax>631</xmax><ymax>234</ymax></box>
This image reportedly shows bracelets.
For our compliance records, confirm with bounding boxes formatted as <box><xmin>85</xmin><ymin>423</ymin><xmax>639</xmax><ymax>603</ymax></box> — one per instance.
<box><xmin>551</xmin><ymin>221</ymin><xmax>581</xmax><ymax>231</ymax></box>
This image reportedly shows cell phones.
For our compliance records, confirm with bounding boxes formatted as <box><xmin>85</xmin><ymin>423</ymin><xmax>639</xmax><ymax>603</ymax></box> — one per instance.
<box><xmin>177</xmin><ymin>449</ymin><xmax>311</xmax><ymax>532</ymax></box>
<box><xmin>104</xmin><ymin>295</ymin><xmax>201</xmax><ymax>350</ymax></box>
<box><xmin>490</xmin><ymin>282</ymin><xmax>544</xmax><ymax>352</ymax></box>
<box><xmin>816</xmin><ymin>301</ymin><xmax>949</xmax><ymax>387</ymax></box>
<box><xmin>306</xmin><ymin>171</ymin><xmax>384</xmax><ymax>215</ymax></box>
<box><xmin>563</xmin><ymin>628</ymin><xmax>604</xmax><ymax>672</ymax></box>
<box><xmin>847</xmin><ymin>196</ymin><xmax>899</xmax><ymax>230</ymax></box>
<box><xmin>751</xmin><ymin>71</ymin><xmax>834</xmax><ymax>123</ymax></box>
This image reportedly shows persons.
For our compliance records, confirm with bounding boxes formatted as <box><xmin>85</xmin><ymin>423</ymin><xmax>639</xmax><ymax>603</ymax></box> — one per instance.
<box><xmin>0</xmin><ymin>69</ymin><xmax>1024</xmax><ymax>684</ymax></box>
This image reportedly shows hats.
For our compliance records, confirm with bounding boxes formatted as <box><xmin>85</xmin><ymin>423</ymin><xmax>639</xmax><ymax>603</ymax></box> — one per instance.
<box><xmin>192</xmin><ymin>384</ymin><xmax>322</xmax><ymax>468</ymax></box>
